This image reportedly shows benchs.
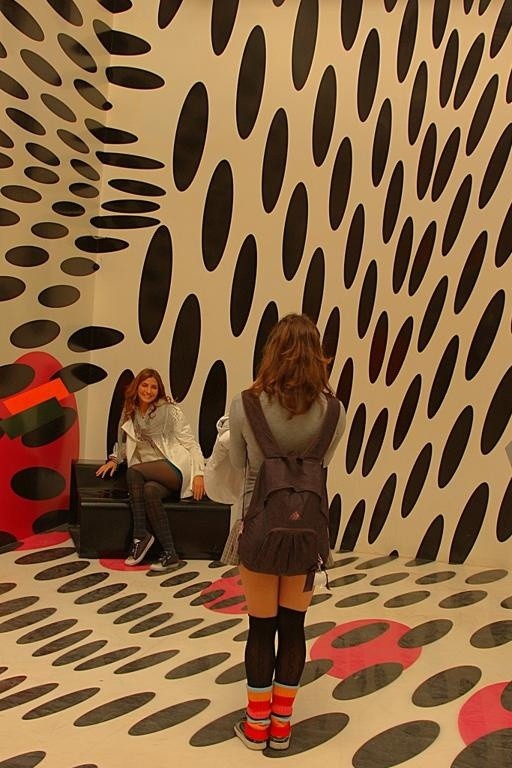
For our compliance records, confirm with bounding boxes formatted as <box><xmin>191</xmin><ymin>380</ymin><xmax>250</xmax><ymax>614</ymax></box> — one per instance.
<box><xmin>68</xmin><ymin>460</ymin><xmax>231</xmax><ymax>561</ymax></box>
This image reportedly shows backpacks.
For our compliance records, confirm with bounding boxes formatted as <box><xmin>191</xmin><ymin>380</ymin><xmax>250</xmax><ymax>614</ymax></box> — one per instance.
<box><xmin>238</xmin><ymin>388</ymin><xmax>341</xmax><ymax>576</ymax></box>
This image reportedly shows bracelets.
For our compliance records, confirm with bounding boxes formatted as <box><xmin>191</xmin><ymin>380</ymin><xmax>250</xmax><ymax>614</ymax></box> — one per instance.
<box><xmin>105</xmin><ymin>458</ymin><xmax>118</xmax><ymax>464</ymax></box>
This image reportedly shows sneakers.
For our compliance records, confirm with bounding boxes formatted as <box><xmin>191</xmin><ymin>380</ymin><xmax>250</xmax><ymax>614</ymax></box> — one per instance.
<box><xmin>125</xmin><ymin>533</ymin><xmax>155</xmax><ymax>566</ymax></box>
<box><xmin>151</xmin><ymin>553</ymin><xmax>179</xmax><ymax>572</ymax></box>
<box><xmin>233</xmin><ymin>720</ymin><xmax>291</xmax><ymax>750</ymax></box>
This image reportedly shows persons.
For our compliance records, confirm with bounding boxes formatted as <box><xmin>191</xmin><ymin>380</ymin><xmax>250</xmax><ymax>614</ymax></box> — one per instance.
<box><xmin>96</xmin><ymin>368</ymin><xmax>206</xmax><ymax>571</ymax></box>
<box><xmin>220</xmin><ymin>313</ymin><xmax>346</xmax><ymax>750</ymax></box>
